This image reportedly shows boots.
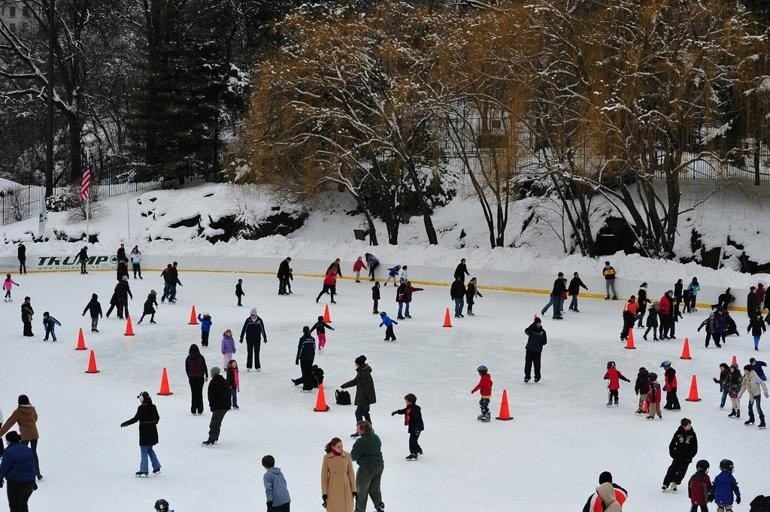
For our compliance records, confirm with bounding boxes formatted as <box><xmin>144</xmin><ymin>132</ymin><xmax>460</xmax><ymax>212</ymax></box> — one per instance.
<box><xmin>744</xmin><ymin>419</ymin><xmax>766</xmax><ymax>427</ymax></box>
<box><xmin>728</xmin><ymin>409</ymin><xmax>740</xmax><ymax>417</ymax></box>
<box><xmin>478</xmin><ymin>412</ymin><xmax>491</xmax><ymax>420</ymax></box>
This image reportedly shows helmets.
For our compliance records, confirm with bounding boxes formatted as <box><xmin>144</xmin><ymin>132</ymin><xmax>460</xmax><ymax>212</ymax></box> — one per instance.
<box><xmin>477</xmin><ymin>365</ymin><xmax>488</xmax><ymax>374</ymax></box>
<box><xmin>696</xmin><ymin>459</ymin><xmax>710</xmax><ymax>472</ymax></box>
<box><xmin>607</xmin><ymin>361</ymin><xmax>616</xmax><ymax>368</ymax></box>
<box><xmin>647</xmin><ymin>372</ymin><xmax>658</xmax><ymax>381</ymax></box>
<box><xmin>660</xmin><ymin>361</ymin><xmax>671</xmax><ymax>368</ymax></box>
<box><xmin>720</xmin><ymin>459</ymin><xmax>734</xmax><ymax>473</ymax></box>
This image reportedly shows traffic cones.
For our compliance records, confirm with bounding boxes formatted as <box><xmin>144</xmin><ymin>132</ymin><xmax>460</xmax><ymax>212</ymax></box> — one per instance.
<box><xmin>439</xmin><ymin>306</ymin><xmax>453</xmax><ymax>328</ymax></box>
<box><xmin>323</xmin><ymin>303</ymin><xmax>332</xmax><ymax>324</ymax></box>
<box><xmin>154</xmin><ymin>365</ymin><xmax>175</xmax><ymax>399</ymax></box>
<box><xmin>185</xmin><ymin>303</ymin><xmax>201</xmax><ymax>325</ymax></box>
<box><xmin>82</xmin><ymin>349</ymin><xmax>100</xmax><ymax>375</ymax></box>
<box><xmin>74</xmin><ymin>327</ymin><xmax>87</xmax><ymax>351</ymax></box>
<box><xmin>495</xmin><ymin>387</ymin><xmax>515</xmax><ymax>422</ymax></box>
<box><xmin>312</xmin><ymin>382</ymin><xmax>331</xmax><ymax>413</ymax></box>
<box><xmin>623</xmin><ymin>327</ymin><xmax>637</xmax><ymax>350</ymax></box>
<box><xmin>731</xmin><ymin>351</ymin><xmax>740</xmax><ymax>365</ymax></box>
<box><xmin>678</xmin><ymin>336</ymin><xmax>692</xmax><ymax>361</ymax></box>
<box><xmin>684</xmin><ymin>373</ymin><xmax>704</xmax><ymax>403</ymax></box>
<box><xmin>121</xmin><ymin>314</ymin><xmax>137</xmax><ymax>336</ymax></box>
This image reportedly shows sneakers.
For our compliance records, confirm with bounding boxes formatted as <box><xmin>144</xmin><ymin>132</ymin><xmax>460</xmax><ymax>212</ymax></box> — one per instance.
<box><xmin>233</xmin><ymin>404</ymin><xmax>239</xmax><ymax>408</ymax></box>
<box><xmin>246</xmin><ymin>366</ymin><xmax>261</xmax><ymax>371</ymax></box>
<box><xmin>662</xmin><ymin>483</ymin><xmax>677</xmax><ymax>491</ymax></box>
<box><xmin>646</xmin><ymin>415</ymin><xmax>662</xmax><ymax>418</ymax></box>
<box><xmin>351</xmin><ymin>432</ymin><xmax>360</xmax><ymax>438</ymax></box>
<box><xmin>406</xmin><ymin>450</ymin><xmax>422</xmax><ymax>459</ymax></box>
<box><xmin>607</xmin><ymin>401</ymin><xmax>619</xmax><ymax>405</ymax></box>
<box><xmin>190</xmin><ymin>408</ymin><xmax>203</xmax><ymax>415</ymax></box>
<box><xmin>202</xmin><ymin>438</ymin><xmax>218</xmax><ymax>444</ymax></box>
<box><xmin>524</xmin><ymin>377</ymin><xmax>540</xmax><ymax>382</ymax></box>
<box><xmin>135</xmin><ymin>467</ymin><xmax>161</xmax><ymax>476</ymax></box>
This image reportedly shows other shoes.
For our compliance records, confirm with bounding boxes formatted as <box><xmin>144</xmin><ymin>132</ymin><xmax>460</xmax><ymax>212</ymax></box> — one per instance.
<box><xmin>279</xmin><ymin>289</ymin><xmax>293</xmax><ymax>295</ymax></box>
<box><xmin>4</xmin><ymin>298</ymin><xmax>7</xmax><ymax>303</ymax></box>
<box><xmin>53</xmin><ymin>338</ymin><xmax>57</xmax><ymax>341</ymax></box>
<box><xmin>354</xmin><ymin>280</ymin><xmax>413</xmax><ymax>342</ymax></box>
<box><xmin>238</xmin><ymin>303</ymin><xmax>243</xmax><ymax>307</ymax></box>
<box><xmin>604</xmin><ymin>294</ymin><xmax>765</xmax><ymax>351</ymax></box>
<box><xmin>316</xmin><ymin>297</ymin><xmax>319</xmax><ymax>303</ymax></box>
<box><xmin>334</xmin><ymin>291</ymin><xmax>337</xmax><ymax>294</ymax></box>
<box><xmin>43</xmin><ymin>337</ymin><xmax>47</xmax><ymax>341</ymax></box>
<box><xmin>79</xmin><ymin>271</ymin><xmax>179</xmax><ymax>332</ymax></box>
<box><xmin>542</xmin><ymin>306</ymin><xmax>581</xmax><ymax>320</ymax></box>
<box><xmin>331</xmin><ymin>300</ymin><xmax>336</xmax><ymax>303</ymax></box>
<box><xmin>7</xmin><ymin>298</ymin><xmax>12</xmax><ymax>303</ymax></box>
<box><xmin>326</xmin><ymin>291</ymin><xmax>331</xmax><ymax>294</ymax></box>
<box><xmin>454</xmin><ymin>311</ymin><xmax>475</xmax><ymax>319</ymax></box>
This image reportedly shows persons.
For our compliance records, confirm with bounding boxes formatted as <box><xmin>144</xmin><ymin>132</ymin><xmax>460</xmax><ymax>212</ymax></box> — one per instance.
<box><xmin>542</xmin><ymin>258</ymin><xmax>770</xmax><ymax>511</ymax></box>
<box><xmin>469</xmin><ymin>365</ymin><xmax>494</xmax><ymax>423</ymax></box>
<box><xmin>521</xmin><ymin>315</ymin><xmax>548</xmax><ymax>383</ymax></box>
<box><xmin>0</xmin><ymin>240</ymin><xmax>484</xmax><ymax>512</ymax></box>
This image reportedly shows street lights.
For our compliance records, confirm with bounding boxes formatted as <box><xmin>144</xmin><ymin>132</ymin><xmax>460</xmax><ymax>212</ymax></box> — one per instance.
<box><xmin>124</xmin><ymin>171</ymin><xmax>137</xmax><ymax>244</ymax></box>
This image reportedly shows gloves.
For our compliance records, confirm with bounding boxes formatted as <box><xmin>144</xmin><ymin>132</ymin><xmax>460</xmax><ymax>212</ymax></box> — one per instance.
<box><xmin>736</xmin><ymin>496</ymin><xmax>741</xmax><ymax>504</ymax></box>
<box><xmin>707</xmin><ymin>493</ymin><xmax>716</xmax><ymax>503</ymax></box>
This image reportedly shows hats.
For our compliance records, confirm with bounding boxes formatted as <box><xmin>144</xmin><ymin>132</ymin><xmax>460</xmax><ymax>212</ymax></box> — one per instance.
<box><xmin>731</xmin><ymin>363</ymin><xmax>738</xmax><ymax>369</ymax></box>
<box><xmin>261</xmin><ymin>455</ymin><xmax>276</xmax><ymax>468</ymax></box>
<box><xmin>154</xmin><ymin>498</ymin><xmax>169</xmax><ymax>512</ymax></box>
<box><xmin>355</xmin><ymin>355</ymin><xmax>367</xmax><ymax>366</ymax></box>
<box><xmin>5</xmin><ymin>431</ymin><xmax>21</xmax><ymax>443</ymax></box>
<box><xmin>137</xmin><ymin>391</ymin><xmax>149</xmax><ymax>398</ymax></box>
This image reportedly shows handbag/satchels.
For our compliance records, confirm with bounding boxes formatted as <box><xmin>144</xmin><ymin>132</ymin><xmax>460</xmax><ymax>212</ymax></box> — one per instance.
<box><xmin>335</xmin><ymin>388</ymin><xmax>352</xmax><ymax>405</ymax></box>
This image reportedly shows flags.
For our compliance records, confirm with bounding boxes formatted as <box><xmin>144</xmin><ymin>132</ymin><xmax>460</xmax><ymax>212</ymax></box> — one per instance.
<box><xmin>80</xmin><ymin>163</ymin><xmax>91</xmax><ymax>202</ymax></box>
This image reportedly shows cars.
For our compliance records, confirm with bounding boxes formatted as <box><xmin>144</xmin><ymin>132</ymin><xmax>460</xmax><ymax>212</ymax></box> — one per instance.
<box><xmin>114</xmin><ymin>166</ymin><xmax>159</xmax><ymax>183</ymax></box>
